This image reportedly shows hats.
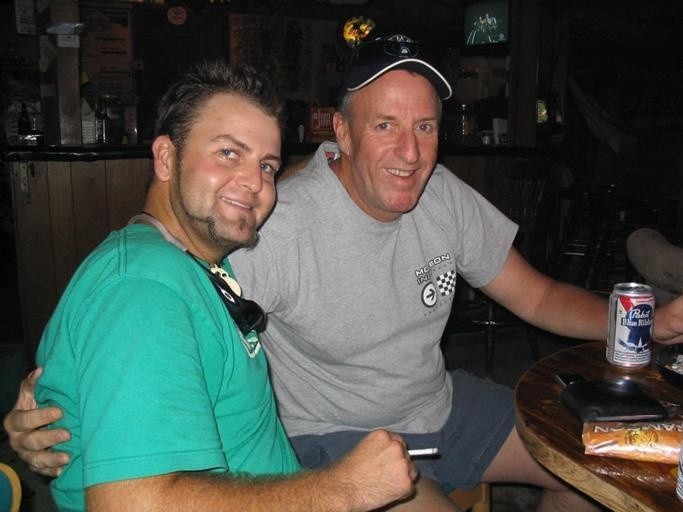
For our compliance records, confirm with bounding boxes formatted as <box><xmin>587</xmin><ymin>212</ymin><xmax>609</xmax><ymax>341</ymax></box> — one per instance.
<box><xmin>341</xmin><ymin>27</ymin><xmax>453</xmax><ymax>102</ymax></box>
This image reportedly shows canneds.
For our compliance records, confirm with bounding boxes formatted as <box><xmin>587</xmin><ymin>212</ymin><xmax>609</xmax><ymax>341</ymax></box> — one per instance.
<box><xmin>605</xmin><ymin>283</ymin><xmax>656</xmax><ymax>371</ymax></box>
<box><xmin>481</xmin><ymin>131</ymin><xmax>494</xmax><ymax>144</ymax></box>
<box><xmin>95</xmin><ymin>95</ymin><xmax>124</xmax><ymax>143</ymax></box>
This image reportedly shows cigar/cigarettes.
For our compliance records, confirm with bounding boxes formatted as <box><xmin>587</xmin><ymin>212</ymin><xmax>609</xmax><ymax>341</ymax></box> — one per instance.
<box><xmin>408</xmin><ymin>446</ymin><xmax>438</xmax><ymax>459</ymax></box>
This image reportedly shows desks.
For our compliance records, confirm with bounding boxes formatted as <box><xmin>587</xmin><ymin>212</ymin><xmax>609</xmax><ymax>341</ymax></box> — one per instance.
<box><xmin>511</xmin><ymin>338</ymin><xmax>682</xmax><ymax>512</ymax></box>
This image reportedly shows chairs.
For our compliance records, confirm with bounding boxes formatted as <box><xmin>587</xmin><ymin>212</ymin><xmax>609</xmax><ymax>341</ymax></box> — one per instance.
<box><xmin>442</xmin><ymin>158</ymin><xmax>661</xmax><ymax>370</ymax></box>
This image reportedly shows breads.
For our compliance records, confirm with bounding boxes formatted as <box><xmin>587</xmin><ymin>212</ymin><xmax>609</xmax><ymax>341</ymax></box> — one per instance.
<box><xmin>583</xmin><ymin>423</ymin><xmax>683</xmax><ymax>463</ymax></box>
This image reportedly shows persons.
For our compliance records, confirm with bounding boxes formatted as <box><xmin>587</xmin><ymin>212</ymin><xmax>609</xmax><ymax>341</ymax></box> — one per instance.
<box><xmin>34</xmin><ymin>55</ymin><xmax>420</xmax><ymax>511</ymax></box>
<box><xmin>3</xmin><ymin>28</ymin><xmax>682</xmax><ymax>512</ymax></box>
